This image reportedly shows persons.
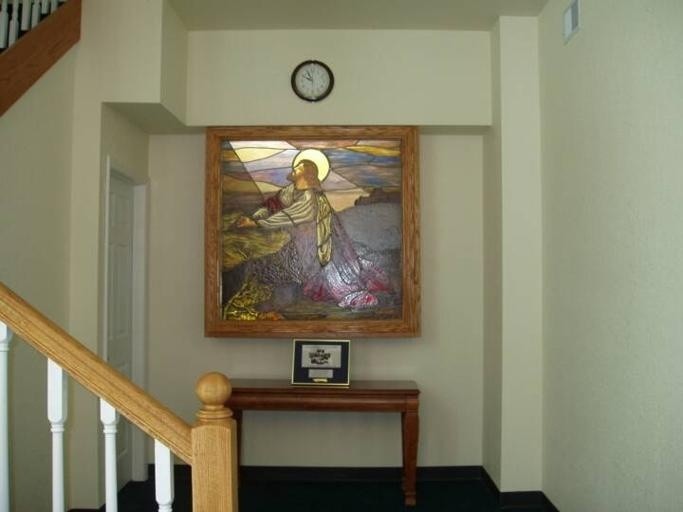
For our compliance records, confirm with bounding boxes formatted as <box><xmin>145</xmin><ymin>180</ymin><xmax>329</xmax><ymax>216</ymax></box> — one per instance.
<box><xmin>231</xmin><ymin>159</ymin><xmax>392</xmax><ymax>312</ymax></box>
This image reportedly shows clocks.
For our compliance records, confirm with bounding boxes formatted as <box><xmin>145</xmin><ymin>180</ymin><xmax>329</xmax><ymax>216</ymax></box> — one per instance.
<box><xmin>291</xmin><ymin>60</ymin><xmax>334</xmax><ymax>101</ymax></box>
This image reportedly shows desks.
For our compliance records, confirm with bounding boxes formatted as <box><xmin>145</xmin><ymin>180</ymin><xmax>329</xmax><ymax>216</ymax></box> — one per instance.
<box><xmin>225</xmin><ymin>379</ymin><xmax>421</xmax><ymax>511</ymax></box>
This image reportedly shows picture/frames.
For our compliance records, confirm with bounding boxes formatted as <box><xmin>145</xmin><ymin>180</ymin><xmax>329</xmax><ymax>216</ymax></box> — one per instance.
<box><xmin>291</xmin><ymin>339</ymin><xmax>351</xmax><ymax>389</ymax></box>
<box><xmin>205</xmin><ymin>124</ymin><xmax>422</xmax><ymax>341</ymax></box>
